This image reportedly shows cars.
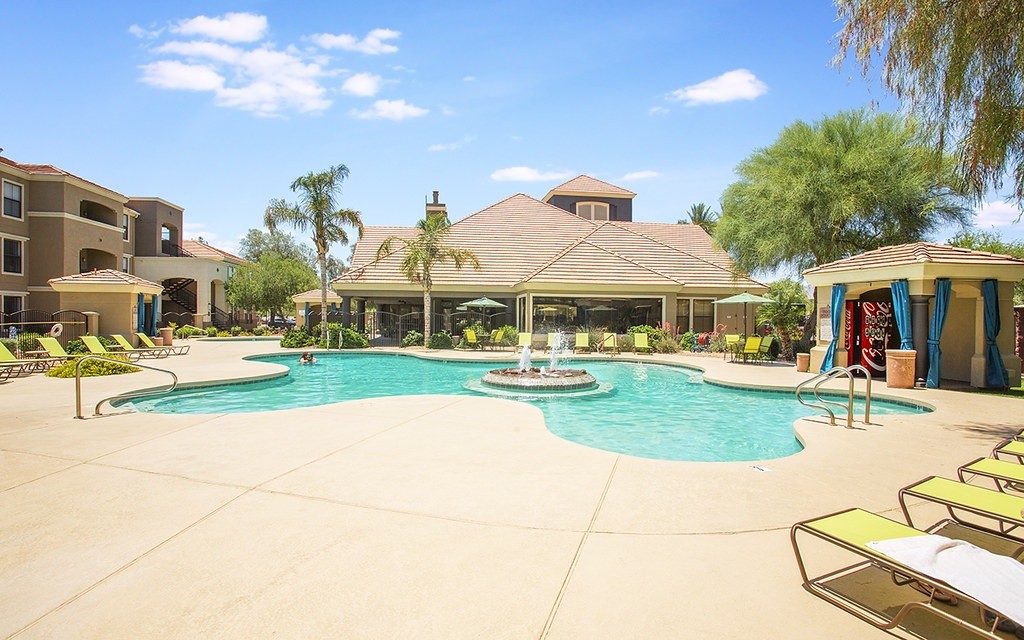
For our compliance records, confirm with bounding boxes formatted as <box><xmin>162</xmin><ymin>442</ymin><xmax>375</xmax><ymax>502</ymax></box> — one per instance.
<box><xmin>268</xmin><ymin>318</ymin><xmax>296</xmax><ymax>328</ymax></box>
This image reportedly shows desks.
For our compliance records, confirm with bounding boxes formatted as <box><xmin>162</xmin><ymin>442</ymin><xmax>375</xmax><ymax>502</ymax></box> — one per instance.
<box><xmin>730</xmin><ymin>341</ymin><xmax>745</xmax><ymax>365</ymax></box>
<box><xmin>474</xmin><ymin>334</ymin><xmax>493</xmax><ymax>351</ymax></box>
<box><xmin>106</xmin><ymin>344</ymin><xmax>125</xmax><ymax>356</ymax></box>
<box><xmin>25</xmin><ymin>350</ymin><xmax>50</xmax><ymax>370</ymax></box>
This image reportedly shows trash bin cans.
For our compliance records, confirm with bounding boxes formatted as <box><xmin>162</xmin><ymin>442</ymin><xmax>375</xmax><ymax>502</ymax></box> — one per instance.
<box><xmin>154</xmin><ymin>338</ymin><xmax>163</xmax><ymax>346</ymax></box>
<box><xmin>159</xmin><ymin>328</ymin><xmax>173</xmax><ymax>346</ymax></box>
<box><xmin>885</xmin><ymin>350</ymin><xmax>917</xmax><ymax>388</ymax></box>
<box><xmin>797</xmin><ymin>353</ymin><xmax>810</xmax><ymax>373</ymax></box>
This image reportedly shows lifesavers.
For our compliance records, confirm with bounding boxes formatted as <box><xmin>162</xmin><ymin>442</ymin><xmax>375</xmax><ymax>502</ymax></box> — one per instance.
<box><xmin>50</xmin><ymin>323</ymin><xmax>63</xmax><ymax>338</ymax></box>
<box><xmin>301</xmin><ymin>354</ymin><xmax>317</xmax><ymax>363</ymax></box>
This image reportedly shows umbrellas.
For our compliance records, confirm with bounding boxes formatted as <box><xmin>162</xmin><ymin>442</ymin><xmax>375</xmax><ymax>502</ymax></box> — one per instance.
<box><xmin>460</xmin><ymin>298</ymin><xmax>508</xmax><ymax>352</ymax></box>
<box><xmin>712</xmin><ymin>291</ymin><xmax>780</xmax><ymax>363</ymax></box>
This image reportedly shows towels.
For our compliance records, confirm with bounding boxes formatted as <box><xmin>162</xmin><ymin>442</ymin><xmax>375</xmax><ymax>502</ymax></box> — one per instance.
<box><xmin>866</xmin><ymin>532</ymin><xmax>1024</xmax><ymax>626</ymax></box>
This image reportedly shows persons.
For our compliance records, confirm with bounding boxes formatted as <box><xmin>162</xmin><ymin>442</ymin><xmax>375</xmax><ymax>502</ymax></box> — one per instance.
<box><xmin>299</xmin><ymin>352</ymin><xmax>316</xmax><ymax>363</ymax></box>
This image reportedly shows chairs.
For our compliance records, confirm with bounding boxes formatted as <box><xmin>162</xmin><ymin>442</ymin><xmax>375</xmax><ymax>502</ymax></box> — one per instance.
<box><xmin>134</xmin><ymin>332</ymin><xmax>190</xmax><ymax>355</ymax></box>
<box><xmin>515</xmin><ymin>332</ymin><xmax>533</xmax><ymax>354</ymax></box>
<box><xmin>0</xmin><ymin>341</ymin><xmax>68</xmax><ymax>381</ymax></box>
<box><xmin>791</xmin><ymin>428</ymin><xmax>1024</xmax><ymax>640</ymax></box>
<box><xmin>544</xmin><ymin>332</ymin><xmax>562</xmax><ymax>355</ymax></box>
<box><xmin>33</xmin><ymin>337</ymin><xmax>84</xmax><ymax>368</ymax></box>
<box><xmin>79</xmin><ymin>335</ymin><xmax>142</xmax><ymax>363</ymax></box>
<box><xmin>461</xmin><ymin>329</ymin><xmax>505</xmax><ymax>351</ymax></box>
<box><xmin>724</xmin><ymin>334</ymin><xmax>776</xmax><ymax>367</ymax></box>
<box><xmin>109</xmin><ymin>334</ymin><xmax>168</xmax><ymax>360</ymax></box>
<box><xmin>572</xmin><ymin>332</ymin><xmax>591</xmax><ymax>354</ymax></box>
<box><xmin>632</xmin><ymin>332</ymin><xmax>653</xmax><ymax>355</ymax></box>
<box><xmin>602</xmin><ymin>333</ymin><xmax>620</xmax><ymax>355</ymax></box>
